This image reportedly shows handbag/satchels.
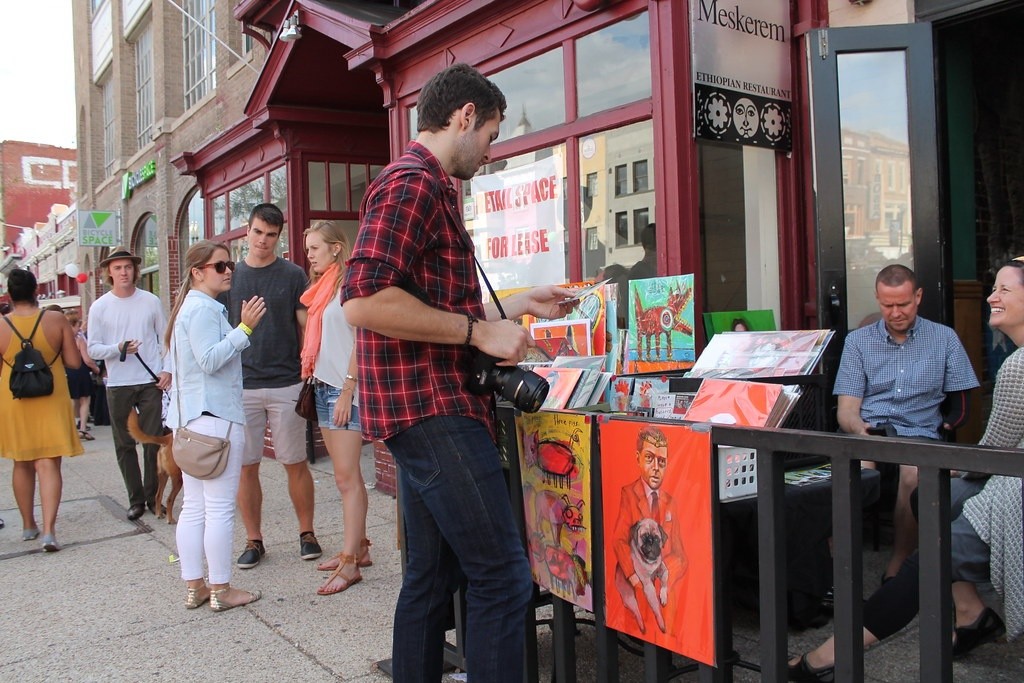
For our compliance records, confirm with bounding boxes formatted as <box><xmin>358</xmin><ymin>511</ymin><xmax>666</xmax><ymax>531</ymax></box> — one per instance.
<box><xmin>295</xmin><ymin>374</ymin><xmax>319</xmax><ymax>422</ymax></box>
<box><xmin>172</xmin><ymin>426</ymin><xmax>231</xmax><ymax>480</ymax></box>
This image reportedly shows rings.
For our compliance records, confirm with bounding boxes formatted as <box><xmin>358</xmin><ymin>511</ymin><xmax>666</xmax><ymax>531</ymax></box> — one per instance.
<box><xmin>332</xmin><ymin>417</ymin><xmax>337</xmax><ymax>420</ymax></box>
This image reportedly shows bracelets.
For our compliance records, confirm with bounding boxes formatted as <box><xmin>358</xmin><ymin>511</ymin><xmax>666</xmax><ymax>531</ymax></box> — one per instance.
<box><xmin>342</xmin><ymin>374</ymin><xmax>357</xmax><ymax>394</ymax></box>
<box><xmin>457</xmin><ymin>311</ymin><xmax>473</xmax><ymax>348</ymax></box>
<box><xmin>238</xmin><ymin>322</ymin><xmax>252</xmax><ymax>336</ymax></box>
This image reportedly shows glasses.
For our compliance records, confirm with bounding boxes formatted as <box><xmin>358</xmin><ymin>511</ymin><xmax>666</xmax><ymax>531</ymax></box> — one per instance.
<box><xmin>198</xmin><ymin>261</ymin><xmax>235</xmax><ymax>274</ymax></box>
<box><xmin>78</xmin><ymin>321</ymin><xmax>82</xmax><ymax>327</ymax></box>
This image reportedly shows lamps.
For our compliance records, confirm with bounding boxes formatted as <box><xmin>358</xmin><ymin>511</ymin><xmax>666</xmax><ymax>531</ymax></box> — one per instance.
<box><xmin>279</xmin><ymin>15</ymin><xmax>303</xmax><ymax>42</ymax></box>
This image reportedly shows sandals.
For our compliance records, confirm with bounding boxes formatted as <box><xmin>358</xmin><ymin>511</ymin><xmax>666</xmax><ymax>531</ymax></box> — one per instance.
<box><xmin>78</xmin><ymin>431</ymin><xmax>95</xmax><ymax>440</ymax></box>
<box><xmin>317</xmin><ymin>553</ymin><xmax>362</xmax><ymax>595</ymax></box>
<box><xmin>210</xmin><ymin>585</ymin><xmax>262</xmax><ymax>612</ymax></box>
<box><xmin>317</xmin><ymin>539</ymin><xmax>372</xmax><ymax>571</ymax></box>
<box><xmin>185</xmin><ymin>582</ymin><xmax>209</xmax><ymax>608</ymax></box>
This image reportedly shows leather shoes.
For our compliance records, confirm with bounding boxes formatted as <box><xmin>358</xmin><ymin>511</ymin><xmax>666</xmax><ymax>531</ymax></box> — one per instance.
<box><xmin>148</xmin><ymin>504</ymin><xmax>167</xmax><ymax>515</ymax></box>
<box><xmin>127</xmin><ymin>502</ymin><xmax>145</xmax><ymax>520</ymax></box>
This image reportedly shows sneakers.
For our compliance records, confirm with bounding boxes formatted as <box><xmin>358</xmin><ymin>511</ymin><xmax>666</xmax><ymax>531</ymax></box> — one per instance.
<box><xmin>300</xmin><ymin>531</ymin><xmax>322</xmax><ymax>562</ymax></box>
<box><xmin>41</xmin><ymin>534</ymin><xmax>63</xmax><ymax>551</ymax></box>
<box><xmin>237</xmin><ymin>538</ymin><xmax>265</xmax><ymax>568</ymax></box>
<box><xmin>22</xmin><ymin>528</ymin><xmax>41</xmax><ymax>540</ymax></box>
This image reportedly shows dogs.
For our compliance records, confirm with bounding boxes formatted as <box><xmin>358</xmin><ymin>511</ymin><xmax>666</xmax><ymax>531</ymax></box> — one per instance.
<box><xmin>128</xmin><ymin>409</ymin><xmax>183</xmax><ymax>525</ymax></box>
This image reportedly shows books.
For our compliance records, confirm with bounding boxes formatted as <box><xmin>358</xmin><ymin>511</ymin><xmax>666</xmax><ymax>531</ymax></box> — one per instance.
<box><xmin>682</xmin><ymin>378</ymin><xmax>802</xmax><ymax>428</ymax></box>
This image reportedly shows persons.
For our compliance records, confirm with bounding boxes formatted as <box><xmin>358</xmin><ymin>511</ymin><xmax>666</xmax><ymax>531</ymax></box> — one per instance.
<box><xmin>787</xmin><ymin>256</ymin><xmax>1024</xmax><ymax>683</ymax></box>
<box><xmin>0</xmin><ymin>301</ymin><xmax>111</xmax><ymax>440</ymax></box>
<box><xmin>732</xmin><ymin>319</ymin><xmax>748</xmax><ymax>332</ymax></box>
<box><xmin>341</xmin><ymin>62</ymin><xmax>579</xmax><ymax>683</ymax></box>
<box><xmin>0</xmin><ymin>269</ymin><xmax>82</xmax><ymax>553</ymax></box>
<box><xmin>215</xmin><ymin>203</ymin><xmax>323</xmax><ymax>569</ymax></box>
<box><xmin>87</xmin><ymin>246</ymin><xmax>173</xmax><ymax>520</ymax></box>
<box><xmin>295</xmin><ymin>220</ymin><xmax>372</xmax><ymax>596</ymax></box>
<box><xmin>822</xmin><ymin>264</ymin><xmax>981</xmax><ymax>601</ymax></box>
<box><xmin>166</xmin><ymin>239</ymin><xmax>267</xmax><ymax>612</ymax></box>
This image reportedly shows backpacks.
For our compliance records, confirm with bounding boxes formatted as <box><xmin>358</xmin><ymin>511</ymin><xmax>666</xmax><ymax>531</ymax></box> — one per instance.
<box><xmin>0</xmin><ymin>310</ymin><xmax>64</xmax><ymax>400</ymax></box>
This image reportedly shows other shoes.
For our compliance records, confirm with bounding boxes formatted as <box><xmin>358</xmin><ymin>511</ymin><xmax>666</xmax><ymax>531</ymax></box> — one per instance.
<box><xmin>77</xmin><ymin>421</ymin><xmax>91</xmax><ymax>431</ymax></box>
<box><xmin>881</xmin><ymin>572</ymin><xmax>895</xmax><ymax>587</ymax></box>
<box><xmin>787</xmin><ymin>653</ymin><xmax>834</xmax><ymax>683</ymax></box>
<box><xmin>952</xmin><ymin>607</ymin><xmax>1007</xmax><ymax>660</ymax></box>
<box><xmin>822</xmin><ymin>585</ymin><xmax>835</xmax><ymax>606</ymax></box>
<box><xmin>0</xmin><ymin>519</ymin><xmax>5</xmax><ymax>529</ymax></box>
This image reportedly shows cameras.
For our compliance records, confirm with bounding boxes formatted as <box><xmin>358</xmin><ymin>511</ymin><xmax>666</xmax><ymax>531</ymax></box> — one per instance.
<box><xmin>463</xmin><ymin>349</ymin><xmax>550</xmax><ymax>413</ymax></box>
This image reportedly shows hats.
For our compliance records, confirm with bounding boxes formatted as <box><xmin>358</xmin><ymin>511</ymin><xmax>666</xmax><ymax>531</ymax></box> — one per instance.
<box><xmin>100</xmin><ymin>245</ymin><xmax>142</xmax><ymax>268</ymax></box>
